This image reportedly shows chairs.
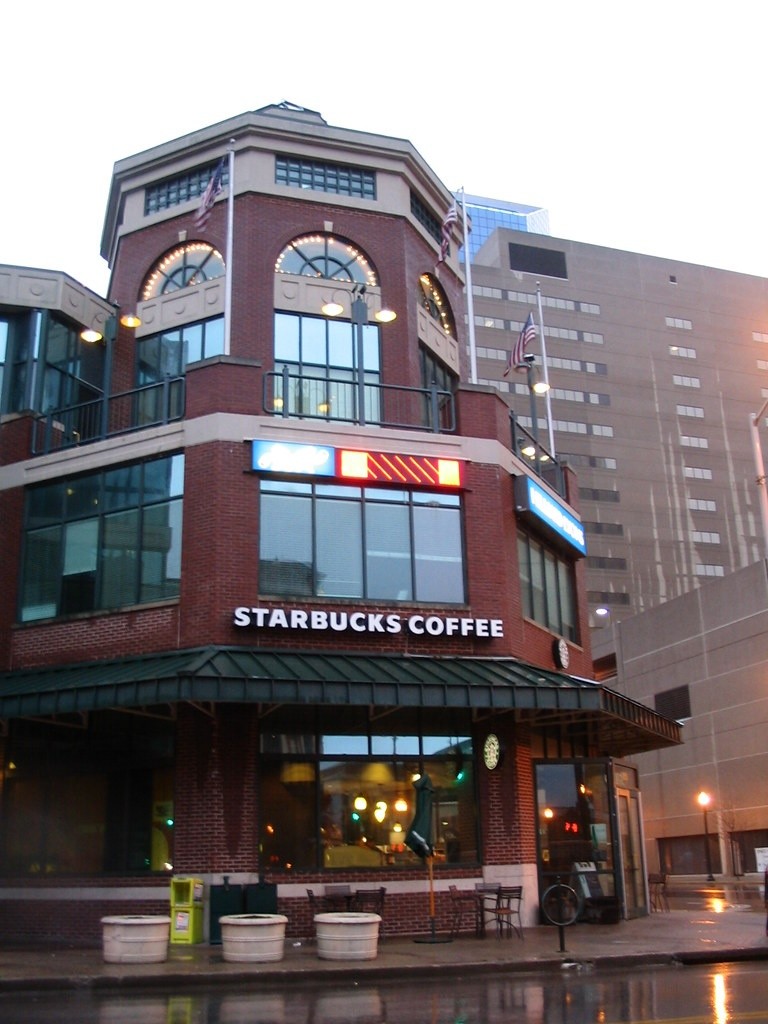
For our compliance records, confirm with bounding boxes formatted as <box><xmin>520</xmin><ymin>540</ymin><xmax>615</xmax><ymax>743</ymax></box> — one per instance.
<box><xmin>475</xmin><ymin>882</ymin><xmax>501</xmax><ymax>936</ymax></box>
<box><xmin>648</xmin><ymin>874</ymin><xmax>670</xmax><ymax>913</ymax></box>
<box><xmin>448</xmin><ymin>885</ymin><xmax>480</xmax><ymax>937</ymax></box>
<box><xmin>305</xmin><ymin>885</ymin><xmax>387</xmax><ymax>946</ymax></box>
<box><xmin>485</xmin><ymin>886</ymin><xmax>523</xmax><ymax>941</ymax></box>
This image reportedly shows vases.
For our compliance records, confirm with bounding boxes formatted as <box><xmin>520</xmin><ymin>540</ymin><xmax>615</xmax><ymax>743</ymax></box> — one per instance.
<box><xmin>101</xmin><ymin>915</ymin><xmax>171</xmax><ymax>964</ymax></box>
<box><xmin>219</xmin><ymin>914</ymin><xmax>288</xmax><ymax>963</ymax></box>
<box><xmin>313</xmin><ymin>911</ymin><xmax>382</xmax><ymax>961</ymax></box>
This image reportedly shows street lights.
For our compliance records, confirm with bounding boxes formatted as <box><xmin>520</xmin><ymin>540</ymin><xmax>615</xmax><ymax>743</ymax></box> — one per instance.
<box><xmin>697</xmin><ymin>792</ymin><xmax>715</xmax><ymax>881</ymax></box>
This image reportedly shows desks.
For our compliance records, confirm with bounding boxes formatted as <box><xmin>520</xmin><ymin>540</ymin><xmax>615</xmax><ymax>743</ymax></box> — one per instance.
<box><xmin>463</xmin><ymin>889</ymin><xmax>503</xmax><ymax>938</ymax></box>
<box><xmin>322</xmin><ymin>893</ymin><xmax>379</xmax><ymax>912</ymax></box>
